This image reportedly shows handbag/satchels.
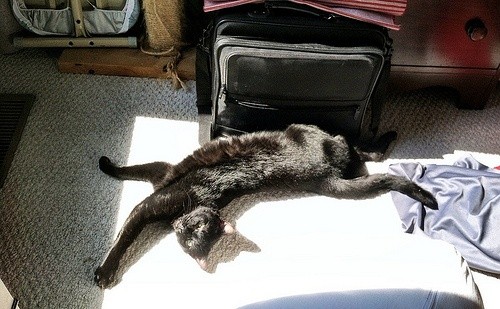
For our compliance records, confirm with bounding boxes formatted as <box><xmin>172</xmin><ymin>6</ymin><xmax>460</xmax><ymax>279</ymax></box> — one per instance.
<box><xmin>195</xmin><ymin>0</ymin><xmax>394</xmax><ymax>147</ymax></box>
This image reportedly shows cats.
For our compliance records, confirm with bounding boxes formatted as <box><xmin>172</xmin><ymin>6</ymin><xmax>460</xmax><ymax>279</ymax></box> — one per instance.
<box><xmin>93</xmin><ymin>123</ymin><xmax>439</xmax><ymax>289</ymax></box>
